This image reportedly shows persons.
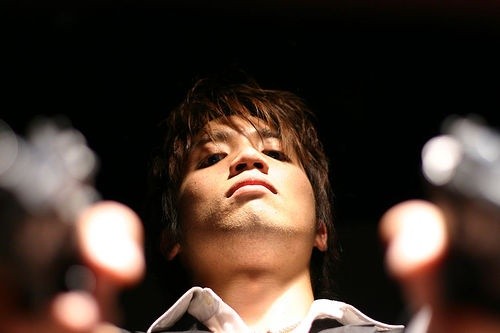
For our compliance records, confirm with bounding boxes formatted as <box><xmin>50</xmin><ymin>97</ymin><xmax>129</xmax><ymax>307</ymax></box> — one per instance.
<box><xmin>0</xmin><ymin>80</ymin><xmax>500</xmax><ymax>333</ymax></box>
